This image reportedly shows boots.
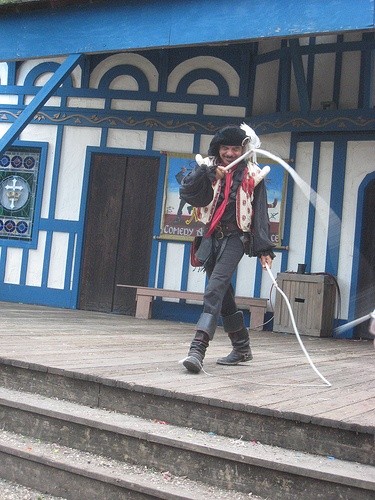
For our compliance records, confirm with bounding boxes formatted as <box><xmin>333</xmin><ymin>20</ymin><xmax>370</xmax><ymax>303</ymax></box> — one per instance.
<box><xmin>217</xmin><ymin>311</ymin><xmax>253</xmax><ymax>365</ymax></box>
<box><xmin>183</xmin><ymin>313</ymin><xmax>215</xmax><ymax>372</ymax></box>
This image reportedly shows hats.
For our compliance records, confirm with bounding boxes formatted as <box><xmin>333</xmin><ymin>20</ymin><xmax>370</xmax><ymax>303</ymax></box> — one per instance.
<box><xmin>208</xmin><ymin>124</ymin><xmax>251</xmax><ymax>156</ymax></box>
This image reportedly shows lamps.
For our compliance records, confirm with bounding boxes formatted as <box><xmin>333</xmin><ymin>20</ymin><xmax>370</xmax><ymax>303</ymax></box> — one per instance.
<box><xmin>320</xmin><ymin>101</ymin><xmax>338</xmax><ymax>111</ymax></box>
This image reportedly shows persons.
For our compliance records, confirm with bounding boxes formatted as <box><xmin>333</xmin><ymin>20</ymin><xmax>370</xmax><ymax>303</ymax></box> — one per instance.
<box><xmin>179</xmin><ymin>126</ymin><xmax>276</xmax><ymax>373</ymax></box>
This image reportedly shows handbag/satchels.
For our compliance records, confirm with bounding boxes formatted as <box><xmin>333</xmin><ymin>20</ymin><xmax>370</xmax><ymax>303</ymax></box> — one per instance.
<box><xmin>190</xmin><ymin>237</ymin><xmax>204</xmax><ymax>268</ymax></box>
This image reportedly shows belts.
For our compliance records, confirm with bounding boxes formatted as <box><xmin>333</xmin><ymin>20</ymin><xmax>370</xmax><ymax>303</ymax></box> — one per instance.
<box><xmin>212</xmin><ymin>230</ymin><xmax>243</xmax><ymax>240</ymax></box>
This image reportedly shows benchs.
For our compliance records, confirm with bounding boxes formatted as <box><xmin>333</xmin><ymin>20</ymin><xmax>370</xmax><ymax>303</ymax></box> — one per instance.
<box><xmin>116</xmin><ymin>280</ymin><xmax>270</xmax><ymax>333</ymax></box>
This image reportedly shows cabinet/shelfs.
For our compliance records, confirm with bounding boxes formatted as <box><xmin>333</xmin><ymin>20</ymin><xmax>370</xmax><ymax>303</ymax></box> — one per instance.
<box><xmin>271</xmin><ymin>270</ymin><xmax>336</xmax><ymax>338</ymax></box>
